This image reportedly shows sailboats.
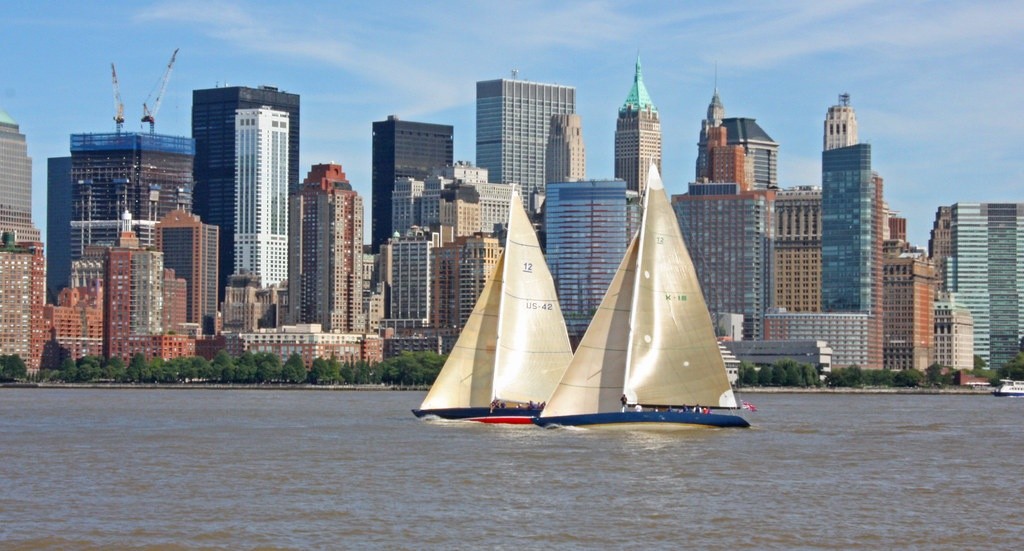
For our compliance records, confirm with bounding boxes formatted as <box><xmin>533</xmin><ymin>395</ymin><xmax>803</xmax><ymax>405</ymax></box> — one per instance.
<box><xmin>410</xmin><ymin>182</ymin><xmax>574</xmax><ymax>428</ymax></box>
<box><xmin>535</xmin><ymin>156</ymin><xmax>750</xmax><ymax>430</ymax></box>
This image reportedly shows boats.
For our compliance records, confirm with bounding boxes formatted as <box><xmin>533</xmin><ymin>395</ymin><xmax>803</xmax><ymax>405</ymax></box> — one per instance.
<box><xmin>990</xmin><ymin>376</ymin><xmax>1024</xmax><ymax>398</ymax></box>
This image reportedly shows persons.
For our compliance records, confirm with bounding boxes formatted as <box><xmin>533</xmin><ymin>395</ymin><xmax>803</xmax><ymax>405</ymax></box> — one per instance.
<box><xmin>619</xmin><ymin>394</ymin><xmax>712</xmax><ymax>414</ymax></box>
<box><xmin>490</xmin><ymin>397</ymin><xmax>546</xmax><ymax>413</ymax></box>
